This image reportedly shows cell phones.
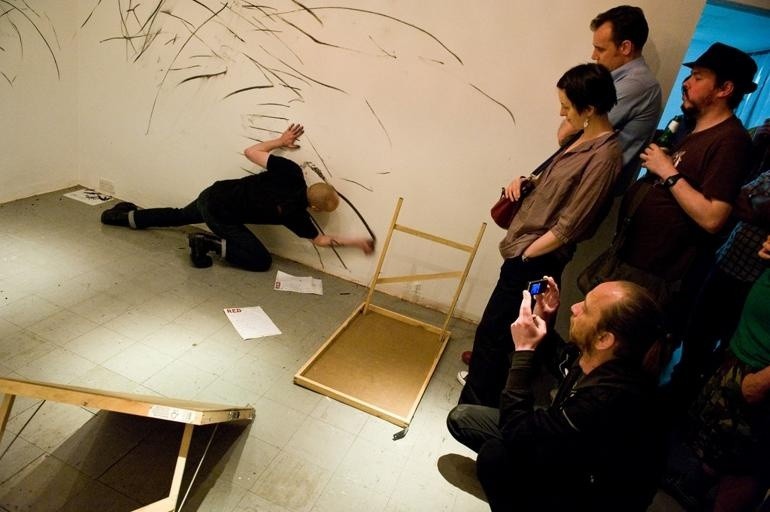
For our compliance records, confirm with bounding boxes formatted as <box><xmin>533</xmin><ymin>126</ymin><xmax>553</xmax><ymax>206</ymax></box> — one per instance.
<box><xmin>527</xmin><ymin>279</ymin><xmax>548</xmax><ymax>295</ymax></box>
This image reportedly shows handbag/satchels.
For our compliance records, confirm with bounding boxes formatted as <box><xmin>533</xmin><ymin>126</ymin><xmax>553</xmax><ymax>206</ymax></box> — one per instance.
<box><xmin>575</xmin><ymin>249</ymin><xmax>672</xmax><ymax>319</ymax></box>
<box><xmin>489</xmin><ymin>175</ymin><xmax>537</xmax><ymax>231</ymax></box>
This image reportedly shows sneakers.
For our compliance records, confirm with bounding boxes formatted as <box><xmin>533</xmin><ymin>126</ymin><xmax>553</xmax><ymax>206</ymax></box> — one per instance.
<box><xmin>457</xmin><ymin>370</ymin><xmax>469</xmax><ymax>386</ymax></box>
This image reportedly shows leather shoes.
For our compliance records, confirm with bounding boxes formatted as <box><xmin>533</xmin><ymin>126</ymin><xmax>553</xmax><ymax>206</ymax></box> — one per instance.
<box><xmin>100</xmin><ymin>200</ymin><xmax>137</xmax><ymax>228</ymax></box>
<box><xmin>188</xmin><ymin>232</ymin><xmax>213</xmax><ymax>268</ymax></box>
<box><xmin>461</xmin><ymin>350</ymin><xmax>472</xmax><ymax>365</ymax></box>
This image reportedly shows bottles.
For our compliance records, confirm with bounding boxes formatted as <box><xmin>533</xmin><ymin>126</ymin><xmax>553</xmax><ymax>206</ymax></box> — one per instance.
<box><xmin>654</xmin><ymin>114</ymin><xmax>682</xmax><ymax>158</ymax></box>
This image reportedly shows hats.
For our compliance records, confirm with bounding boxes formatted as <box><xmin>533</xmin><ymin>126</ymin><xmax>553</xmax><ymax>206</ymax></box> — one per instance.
<box><xmin>682</xmin><ymin>42</ymin><xmax>759</xmax><ymax>96</ymax></box>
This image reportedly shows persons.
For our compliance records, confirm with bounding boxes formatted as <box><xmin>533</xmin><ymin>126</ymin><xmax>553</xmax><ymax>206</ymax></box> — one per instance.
<box><xmin>100</xmin><ymin>122</ymin><xmax>375</xmax><ymax>273</ymax></box>
